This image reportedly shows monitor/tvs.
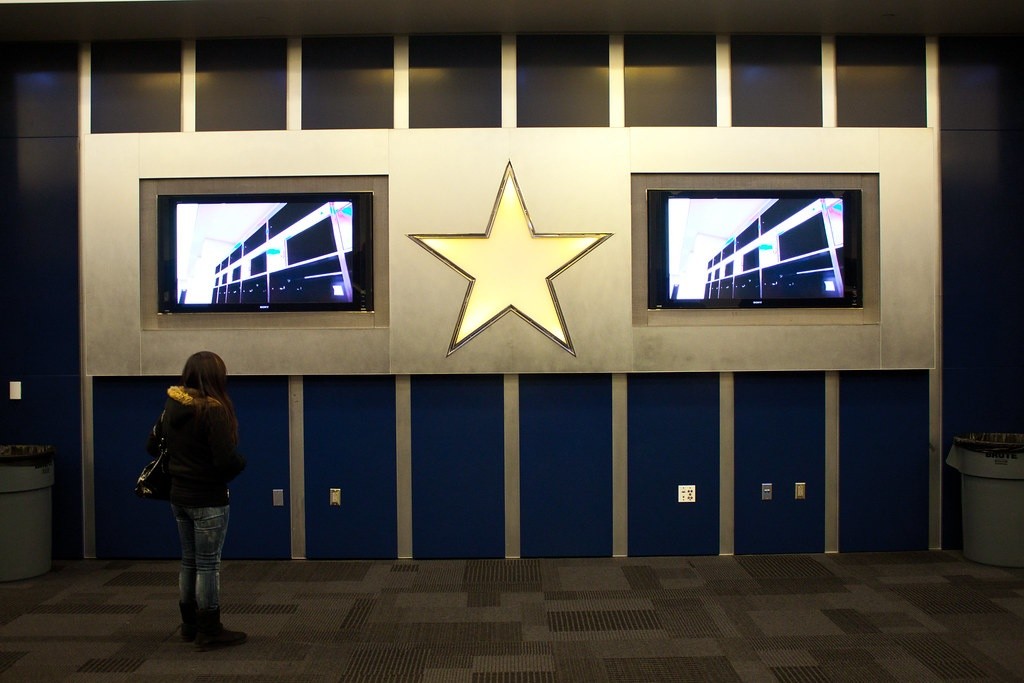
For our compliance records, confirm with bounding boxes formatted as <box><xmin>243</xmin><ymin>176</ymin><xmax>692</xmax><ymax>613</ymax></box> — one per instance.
<box><xmin>157</xmin><ymin>190</ymin><xmax>374</xmax><ymax>313</ymax></box>
<box><xmin>647</xmin><ymin>190</ymin><xmax>863</xmax><ymax>309</ymax></box>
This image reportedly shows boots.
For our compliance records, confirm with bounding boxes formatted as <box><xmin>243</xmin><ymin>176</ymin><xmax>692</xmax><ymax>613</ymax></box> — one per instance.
<box><xmin>179</xmin><ymin>601</ymin><xmax>248</xmax><ymax>653</ymax></box>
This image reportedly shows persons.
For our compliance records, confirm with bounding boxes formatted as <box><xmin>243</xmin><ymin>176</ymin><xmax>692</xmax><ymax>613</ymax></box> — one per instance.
<box><xmin>145</xmin><ymin>351</ymin><xmax>249</xmax><ymax>652</ymax></box>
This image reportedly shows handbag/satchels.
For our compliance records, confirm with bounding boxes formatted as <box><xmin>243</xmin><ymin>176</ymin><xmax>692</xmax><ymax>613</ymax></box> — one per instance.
<box><xmin>135</xmin><ymin>425</ymin><xmax>173</xmax><ymax>506</ymax></box>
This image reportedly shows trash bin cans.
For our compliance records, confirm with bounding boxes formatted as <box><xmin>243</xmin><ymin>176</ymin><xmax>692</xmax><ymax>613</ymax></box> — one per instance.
<box><xmin>944</xmin><ymin>433</ymin><xmax>1024</xmax><ymax>568</ymax></box>
<box><xmin>0</xmin><ymin>445</ymin><xmax>55</xmax><ymax>582</ymax></box>
